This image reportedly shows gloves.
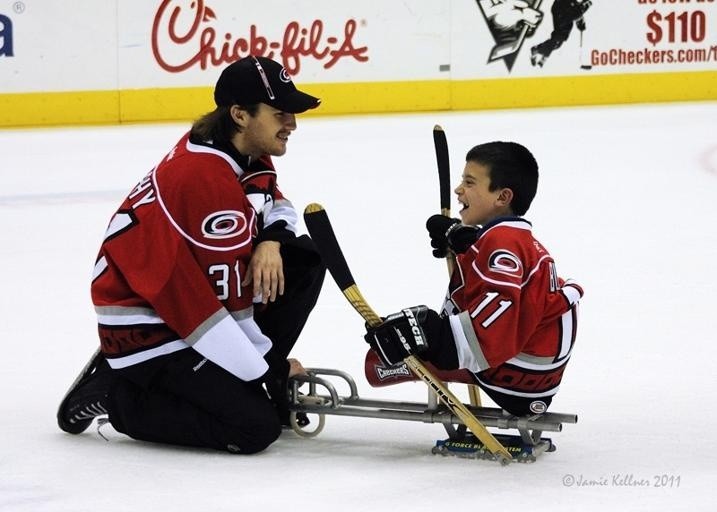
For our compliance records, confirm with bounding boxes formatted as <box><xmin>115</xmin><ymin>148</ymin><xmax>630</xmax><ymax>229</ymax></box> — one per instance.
<box><xmin>212</xmin><ymin>51</ymin><xmax>323</xmax><ymax>116</ymax></box>
<box><xmin>424</xmin><ymin>212</ymin><xmax>481</xmax><ymax>261</ymax></box>
<box><xmin>363</xmin><ymin>303</ymin><xmax>460</xmax><ymax>376</ymax></box>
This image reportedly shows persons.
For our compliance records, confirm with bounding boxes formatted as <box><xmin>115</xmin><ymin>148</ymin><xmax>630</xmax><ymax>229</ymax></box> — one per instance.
<box><xmin>56</xmin><ymin>55</ymin><xmax>328</xmax><ymax>455</ymax></box>
<box><xmin>363</xmin><ymin>140</ymin><xmax>585</xmax><ymax>418</ymax></box>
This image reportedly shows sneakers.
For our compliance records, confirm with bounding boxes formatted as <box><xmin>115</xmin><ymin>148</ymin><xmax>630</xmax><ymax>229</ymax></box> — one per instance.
<box><xmin>56</xmin><ymin>343</ymin><xmax>114</xmax><ymax>444</ymax></box>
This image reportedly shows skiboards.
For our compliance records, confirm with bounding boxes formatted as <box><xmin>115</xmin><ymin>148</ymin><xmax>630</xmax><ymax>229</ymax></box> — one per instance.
<box><xmin>303</xmin><ymin>203</ymin><xmax>516</xmax><ymax>466</ymax></box>
<box><xmin>487</xmin><ymin>1</ymin><xmax>541</xmax><ymax>62</ymax></box>
<box><xmin>433</xmin><ymin>124</ymin><xmax>482</xmax><ymax>407</ymax></box>
